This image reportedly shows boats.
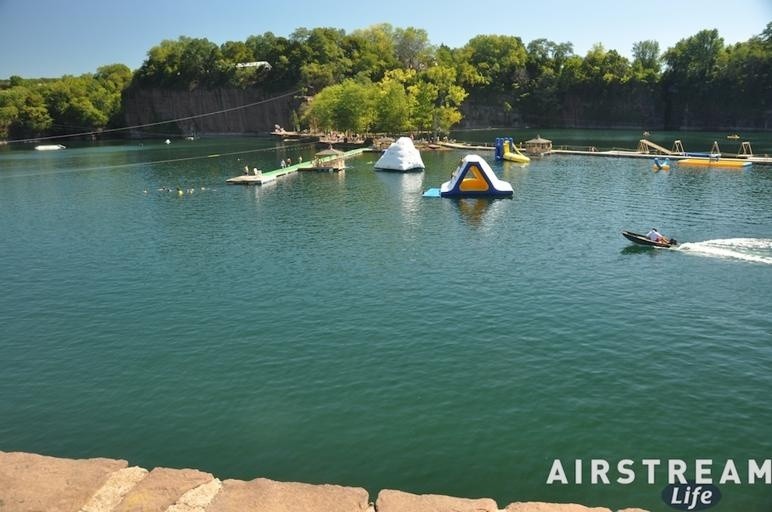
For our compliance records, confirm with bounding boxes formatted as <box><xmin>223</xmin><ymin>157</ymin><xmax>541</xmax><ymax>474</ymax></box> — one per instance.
<box><xmin>619</xmin><ymin>224</ymin><xmax>677</xmax><ymax>252</ymax></box>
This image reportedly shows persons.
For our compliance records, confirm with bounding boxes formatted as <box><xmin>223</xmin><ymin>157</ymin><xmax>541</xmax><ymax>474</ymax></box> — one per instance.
<box><xmin>142</xmin><ymin>183</ymin><xmax>208</xmax><ymax>196</ymax></box>
<box><xmin>280</xmin><ymin>156</ymin><xmax>291</xmax><ymax>169</ymax></box>
<box><xmin>243</xmin><ymin>164</ymin><xmax>262</xmax><ymax>176</ymax></box>
<box><xmin>298</xmin><ymin>156</ymin><xmax>303</xmax><ymax>163</ymax></box>
<box><xmin>430</xmin><ymin>135</ymin><xmax>457</xmax><ymax>143</ymax></box>
<box><xmin>646</xmin><ymin>228</ymin><xmax>668</xmax><ymax>243</ymax></box>
